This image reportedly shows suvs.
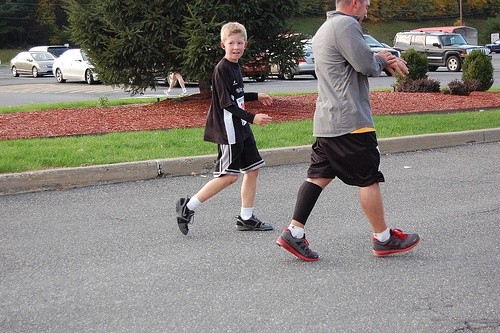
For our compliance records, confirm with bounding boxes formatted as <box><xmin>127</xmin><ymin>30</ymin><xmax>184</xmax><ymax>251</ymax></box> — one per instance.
<box><xmin>393</xmin><ymin>29</ymin><xmax>493</xmax><ymax>72</ymax></box>
<box><xmin>362</xmin><ymin>33</ymin><xmax>402</xmax><ymax>76</ymax></box>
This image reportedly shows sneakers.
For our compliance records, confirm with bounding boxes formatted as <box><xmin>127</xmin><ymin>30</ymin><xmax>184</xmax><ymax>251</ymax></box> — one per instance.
<box><xmin>372</xmin><ymin>228</ymin><xmax>419</xmax><ymax>257</ymax></box>
<box><xmin>236</xmin><ymin>214</ymin><xmax>273</xmax><ymax>231</ymax></box>
<box><xmin>176</xmin><ymin>196</ymin><xmax>194</xmax><ymax>236</ymax></box>
<box><xmin>276</xmin><ymin>228</ymin><xmax>319</xmax><ymax>261</ymax></box>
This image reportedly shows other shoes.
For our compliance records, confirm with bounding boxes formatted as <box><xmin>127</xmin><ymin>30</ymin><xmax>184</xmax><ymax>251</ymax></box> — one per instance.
<box><xmin>163</xmin><ymin>89</ymin><xmax>171</xmax><ymax>96</ymax></box>
<box><xmin>179</xmin><ymin>92</ymin><xmax>188</xmax><ymax>98</ymax></box>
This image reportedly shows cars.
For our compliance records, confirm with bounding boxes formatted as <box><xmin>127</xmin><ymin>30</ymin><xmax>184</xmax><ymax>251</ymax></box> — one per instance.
<box><xmin>256</xmin><ymin>32</ymin><xmax>317</xmax><ymax>79</ymax></box>
<box><xmin>10</xmin><ymin>51</ymin><xmax>58</xmax><ymax>78</ymax></box>
<box><xmin>240</xmin><ymin>51</ymin><xmax>271</xmax><ymax>81</ymax></box>
<box><xmin>486</xmin><ymin>38</ymin><xmax>500</xmax><ymax>53</ymax></box>
<box><xmin>53</xmin><ymin>49</ymin><xmax>103</xmax><ymax>85</ymax></box>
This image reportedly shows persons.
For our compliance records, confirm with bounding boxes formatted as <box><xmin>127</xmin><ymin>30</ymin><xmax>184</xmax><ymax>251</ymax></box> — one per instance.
<box><xmin>176</xmin><ymin>22</ymin><xmax>275</xmax><ymax>235</ymax></box>
<box><xmin>163</xmin><ymin>71</ymin><xmax>188</xmax><ymax>96</ymax></box>
<box><xmin>276</xmin><ymin>0</ymin><xmax>420</xmax><ymax>260</ymax></box>
